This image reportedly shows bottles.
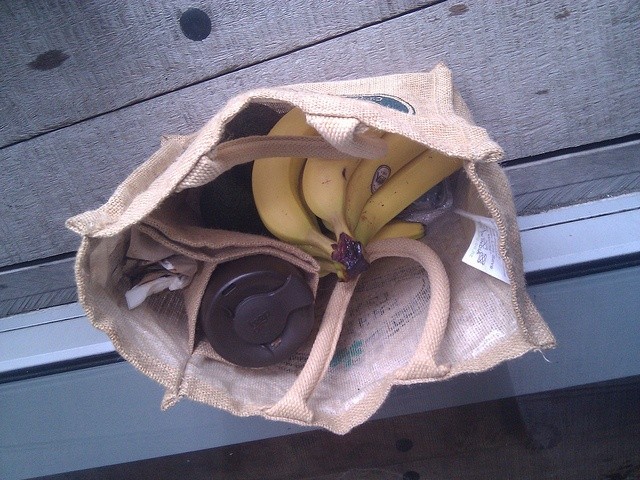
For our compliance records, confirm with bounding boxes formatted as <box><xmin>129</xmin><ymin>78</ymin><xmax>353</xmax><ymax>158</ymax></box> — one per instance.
<box><xmin>197</xmin><ymin>253</ymin><xmax>315</xmax><ymax>368</ymax></box>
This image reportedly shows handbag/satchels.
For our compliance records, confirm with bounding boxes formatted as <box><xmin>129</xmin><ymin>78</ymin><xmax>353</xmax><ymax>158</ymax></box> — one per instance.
<box><xmin>65</xmin><ymin>64</ymin><xmax>557</xmax><ymax>435</ymax></box>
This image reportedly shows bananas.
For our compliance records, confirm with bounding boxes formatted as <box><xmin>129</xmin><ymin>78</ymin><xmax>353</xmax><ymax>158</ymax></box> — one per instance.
<box><xmin>250</xmin><ymin>105</ymin><xmax>465</xmax><ymax>283</ymax></box>
